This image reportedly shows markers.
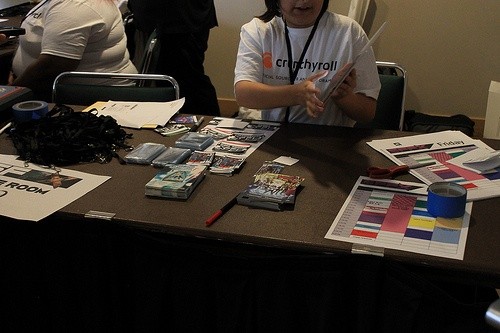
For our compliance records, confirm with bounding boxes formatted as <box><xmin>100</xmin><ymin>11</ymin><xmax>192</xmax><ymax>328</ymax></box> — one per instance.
<box><xmin>189</xmin><ymin>116</ymin><xmax>205</xmax><ymax>132</ymax></box>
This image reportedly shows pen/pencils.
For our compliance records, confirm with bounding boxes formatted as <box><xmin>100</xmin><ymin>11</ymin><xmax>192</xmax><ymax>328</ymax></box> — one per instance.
<box><xmin>0</xmin><ymin>122</ymin><xmax>11</xmax><ymax>134</ymax></box>
<box><xmin>205</xmin><ymin>192</ymin><xmax>243</xmax><ymax>226</ymax></box>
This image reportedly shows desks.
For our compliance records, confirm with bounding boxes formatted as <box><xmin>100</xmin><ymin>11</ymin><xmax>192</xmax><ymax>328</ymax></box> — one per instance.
<box><xmin>0</xmin><ymin>103</ymin><xmax>500</xmax><ymax>333</ymax></box>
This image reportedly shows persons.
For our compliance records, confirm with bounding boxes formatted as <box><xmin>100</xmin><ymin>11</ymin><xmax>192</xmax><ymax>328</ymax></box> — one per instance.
<box><xmin>235</xmin><ymin>0</ymin><xmax>381</xmax><ymax>128</ymax></box>
<box><xmin>126</xmin><ymin>0</ymin><xmax>221</xmax><ymax>117</ymax></box>
<box><xmin>8</xmin><ymin>0</ymin><xmax>140</xmax><ymax>87</ymax></box>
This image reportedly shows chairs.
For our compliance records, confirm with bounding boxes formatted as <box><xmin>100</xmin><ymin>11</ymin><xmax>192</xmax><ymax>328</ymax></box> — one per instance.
<box><xmin>136</xmin><ymin>26</ymin><xmax>163</xmax><ymax>89</ymax></box>
<box><xmin>52</xmin><ymin>72</ymin><xmax>179</xmax><ymax>106</ymax></box>
<box><xmin>375</xmin><ymin>59</ymin><xmax>408</xmax><ymax>132</ymax></box>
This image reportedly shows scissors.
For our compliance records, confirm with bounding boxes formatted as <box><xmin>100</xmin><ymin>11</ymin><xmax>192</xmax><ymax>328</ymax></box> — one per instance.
<box><xmin>366</xmin><ymin>161</ymin><xmax>437</xmax><ymax>180</ymax></box>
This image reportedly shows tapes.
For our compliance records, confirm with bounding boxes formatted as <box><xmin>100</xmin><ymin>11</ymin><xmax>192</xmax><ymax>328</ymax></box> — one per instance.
<box><xmin>11</xmin><ymin>100</ymin><xmax>50</xmax><ymax>122</ymax></box>
<box><xmin>425</xmin><ymin>181</ymin><xmax>468</xmax><ymax>219</ymax></box>
